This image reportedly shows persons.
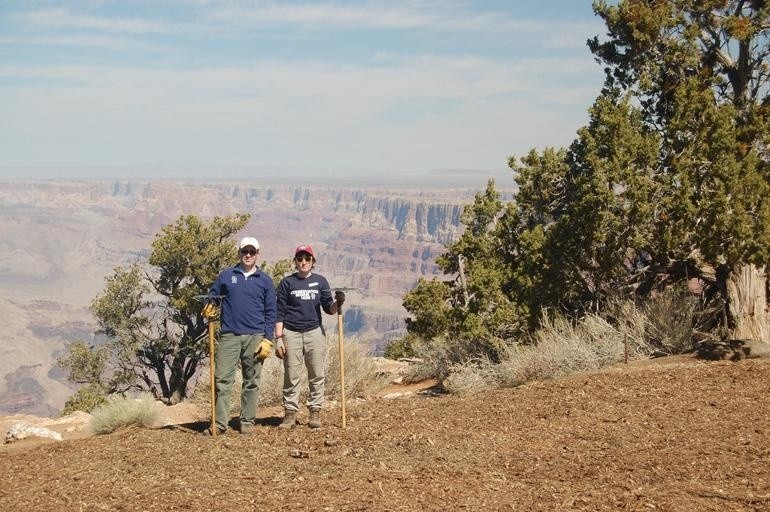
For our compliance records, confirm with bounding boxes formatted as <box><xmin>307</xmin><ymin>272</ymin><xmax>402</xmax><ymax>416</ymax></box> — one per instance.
<box><xmin>274</xmin><ymin>243</ymin><xmax>345</xmax><ymax>430</ymax></box>
<box><xmin>201</xmin><ymin>236</ymin><xmax>277</xmax><ymax>437</ymax></box>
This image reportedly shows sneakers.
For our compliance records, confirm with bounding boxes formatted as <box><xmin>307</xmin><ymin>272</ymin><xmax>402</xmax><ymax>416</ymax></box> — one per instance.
<box><xmin>241</xmin><ymin>424</ymin><xmax>252</xmax><ymax>433</ymax></box>
<box><xmin>202</xmin><ymin>426</ymin><xmax>223</xmax><ymax>435</ymax></box>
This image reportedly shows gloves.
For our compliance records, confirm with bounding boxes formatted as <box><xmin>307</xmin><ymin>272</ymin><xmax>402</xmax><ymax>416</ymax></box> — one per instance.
<box><xmin>255</xmin><ymin>339</ymin><xmax>273</xmax><ymax>360</ymax></box>
<box><xmin>275</xmin><ymin>338</ymin><xmax>286</xmax><ymax>358</ymax></box>
<box><xmin>335</xmin><ymin>291</ymin><xmax>345</xmax><ymax>306</ymax></box>
<box><xmin>202</xmin><ymin>304</ymin><xmax>218</xmax><ymax>318</ymax></box>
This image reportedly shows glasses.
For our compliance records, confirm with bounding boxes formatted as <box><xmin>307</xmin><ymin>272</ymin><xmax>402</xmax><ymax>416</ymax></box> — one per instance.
<box><xmin>240</xmin><ymin>249</ymin><xmax>256</xmax><ymax>255</ymax></box>
<box><xmin>297</xmin><ymin>258</ymin><xmax>311</xmax><ymax>262</ymax></box>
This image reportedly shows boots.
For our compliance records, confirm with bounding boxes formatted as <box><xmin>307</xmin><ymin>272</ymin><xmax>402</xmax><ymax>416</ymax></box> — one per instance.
<box><xmin>309</xmin><ymin>408</ymin><xmax>321</xmax><ymax>428</ymax></box>
<box><xmin>279</xmin><ymin>410</ymin><xmax>295</xmax><ymax>428</ymax></box>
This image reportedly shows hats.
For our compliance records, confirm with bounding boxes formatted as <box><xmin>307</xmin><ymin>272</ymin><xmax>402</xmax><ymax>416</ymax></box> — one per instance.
<box><xmin>239</xmin><ymin>237</ymin><xmax>260</xmax><ymax>253</ymax></box>
<box><xmin>295</xmin><ymin>245</ymin><xmax>313</xmax><ymax>257</ymax></box>
